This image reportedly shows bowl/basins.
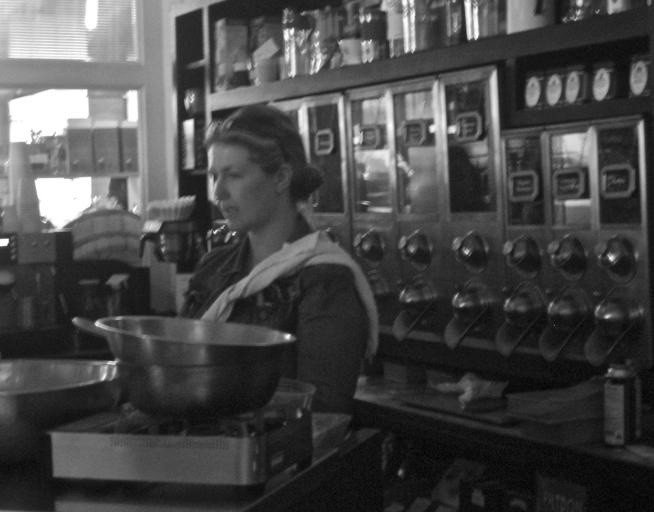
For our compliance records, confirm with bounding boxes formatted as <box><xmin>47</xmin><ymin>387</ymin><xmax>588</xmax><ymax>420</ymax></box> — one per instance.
<box><xmin>0</xmin><ymin>357</ymin><xmax>124</xmax><ymax>471</ymax></box>
<box><xmin>68</xmin><ymin>313</ymin><xmax>299</xmax><ymax>416</ymax></box>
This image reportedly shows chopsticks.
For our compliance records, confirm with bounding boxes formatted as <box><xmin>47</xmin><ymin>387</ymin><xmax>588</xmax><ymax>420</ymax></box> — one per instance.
<box><xmin>146</xmin><ymin>194</ymin><xmax>196</xmax><ymax>223</ymax></box>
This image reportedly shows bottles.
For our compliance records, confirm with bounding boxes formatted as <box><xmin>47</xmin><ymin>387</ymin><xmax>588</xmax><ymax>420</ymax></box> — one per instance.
<box><xmin>282</xmin><ymin>0</ymin><xmax>555</xmax><ymax>81</ymax></box>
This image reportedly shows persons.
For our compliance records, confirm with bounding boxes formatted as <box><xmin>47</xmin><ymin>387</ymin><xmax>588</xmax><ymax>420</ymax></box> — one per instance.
<box><xmin>170</xmin><ymin>103</ymin><xmax>369</xmax><ymax>413</ymax></box>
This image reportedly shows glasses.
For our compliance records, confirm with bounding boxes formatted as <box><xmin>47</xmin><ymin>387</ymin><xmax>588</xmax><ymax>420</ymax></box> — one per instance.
<box><xmin>203</xmin><ymin>120</ymin><xmax>242</xmax><ymax>142</ymax></box>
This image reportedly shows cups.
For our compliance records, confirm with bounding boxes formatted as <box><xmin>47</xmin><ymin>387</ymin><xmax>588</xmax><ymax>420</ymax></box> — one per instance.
<box><xmin>247</xmin><ymin>60</ymin><xmax>280</xmax><ymax>87</ymax></box>
<box><xmin>2</xmin><ymin>141</ymin><xmax>41</xmax><ymax>233</ymax></box>
